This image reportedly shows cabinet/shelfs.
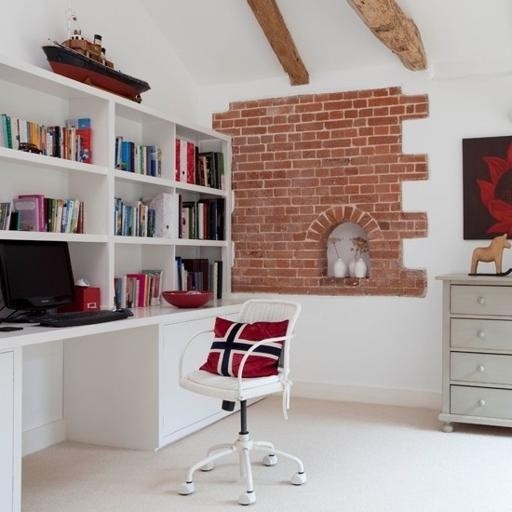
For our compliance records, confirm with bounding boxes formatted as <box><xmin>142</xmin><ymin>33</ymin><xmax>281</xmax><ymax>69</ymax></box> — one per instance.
<box><xmin>0</xmin><ymin>56</ymin><xmax>232</xmax><ymax>512</ymax></box>
<box><xmin>434</xmin><ymin>272</ymin><xmax>512</xmax><ymax>432</ymax></box>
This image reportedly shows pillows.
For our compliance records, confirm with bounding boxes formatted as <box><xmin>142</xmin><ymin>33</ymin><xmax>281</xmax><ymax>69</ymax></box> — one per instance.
<box><xmin>199</xmin><ymin>316</ymin><xmax>289</xmax><ymax>378</ymax></box>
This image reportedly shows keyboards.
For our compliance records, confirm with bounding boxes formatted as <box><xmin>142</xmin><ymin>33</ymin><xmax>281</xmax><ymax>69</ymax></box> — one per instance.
<box><xmin>40</xmin><ymin>309</ymin><xmax>128</xmax><ymax>327</ymax></box>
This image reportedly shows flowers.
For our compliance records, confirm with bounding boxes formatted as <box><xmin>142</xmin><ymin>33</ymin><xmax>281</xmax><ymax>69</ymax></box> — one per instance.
<box><xmin>328</xmin><ymin>237</ymin><xmax>343</xmax><ymax>258</ymax></box>
<box><xmin>350</xmin><ymin>237</ymin><xmax>369</xmax><ymax>258</ymax></box>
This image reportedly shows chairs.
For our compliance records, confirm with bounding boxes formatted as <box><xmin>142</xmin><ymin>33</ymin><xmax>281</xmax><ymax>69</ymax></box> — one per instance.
<box><xmin>178</xmin><ymin>300</ymin><xmax>305</xmax><ymax>504</ymax></box>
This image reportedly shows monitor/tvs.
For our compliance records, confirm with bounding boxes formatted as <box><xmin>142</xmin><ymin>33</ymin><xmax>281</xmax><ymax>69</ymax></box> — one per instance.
<box><xmin>0</xmin><ymin>240</ymin><xmax>76</xmax><ymax>323</ymax></box>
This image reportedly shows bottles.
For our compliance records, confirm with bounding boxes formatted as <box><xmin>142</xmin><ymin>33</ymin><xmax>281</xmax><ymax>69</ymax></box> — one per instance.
<box><xmin>355</xmin><ymin>257</ymin><xmax>367</xmax><ymax>278</ymax></box>
<box><xmin>348</xmin><ymin>258</ymin><xmax>355</xmax><ymax>278</ymax></box>
<box><xmin>334</xmin><ymin>257</ymin><xmax>347</xmax><ymax>278</ymax></box>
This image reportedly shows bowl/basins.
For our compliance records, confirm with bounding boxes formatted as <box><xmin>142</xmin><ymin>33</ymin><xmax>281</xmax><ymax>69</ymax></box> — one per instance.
<box><xmin>162</xmin><ymin>290</ymin><xmax>215</xmax><ymax>308</ymax></box>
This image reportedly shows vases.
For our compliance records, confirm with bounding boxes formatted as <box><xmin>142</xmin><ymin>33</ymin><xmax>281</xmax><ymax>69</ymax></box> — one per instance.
<box><xmin>335</xmin><ymin>259</ymin><xmax>346</xmax><ymax>278</ymax></box>
<box><xmin>349</xmin><ymin>258</ymin><xmax>368</xmax><ymax>278</ymax></box>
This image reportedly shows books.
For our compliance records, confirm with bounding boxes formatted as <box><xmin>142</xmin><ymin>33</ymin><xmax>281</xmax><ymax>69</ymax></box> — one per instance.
<box><xmin>175</xmin><ymin>136</ymin><xmax>224</xmax><ymax>300</ymax></box>
<box><xmin>0</xmin><ymin>191</ymin><xmax>85</xmax><ymax>234</ymax></box>
<box><xmin>113</xmin><ymin>197</ymin><xmax>157</xmax><ymax>237</ymax></box>
<box><xmin>0</xmin><ymin>111</ymin><xmax>93</xmax><ymax>164</ymax></box>
<box><xmin>115</xmin><ymin>135</ymin><xmax>162</xmax><ymax>177</ymax></box>
<box><xmin>114</xmin><ymin>267</ymin><xmax>163</xmax><ymax>310</ymax></box>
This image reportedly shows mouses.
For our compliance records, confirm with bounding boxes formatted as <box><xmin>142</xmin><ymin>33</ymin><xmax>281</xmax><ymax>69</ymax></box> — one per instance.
<box><xmin>115</xmin><ymin>308</ymin><xmax>133</xmax><ymax>316</ymax></box>
